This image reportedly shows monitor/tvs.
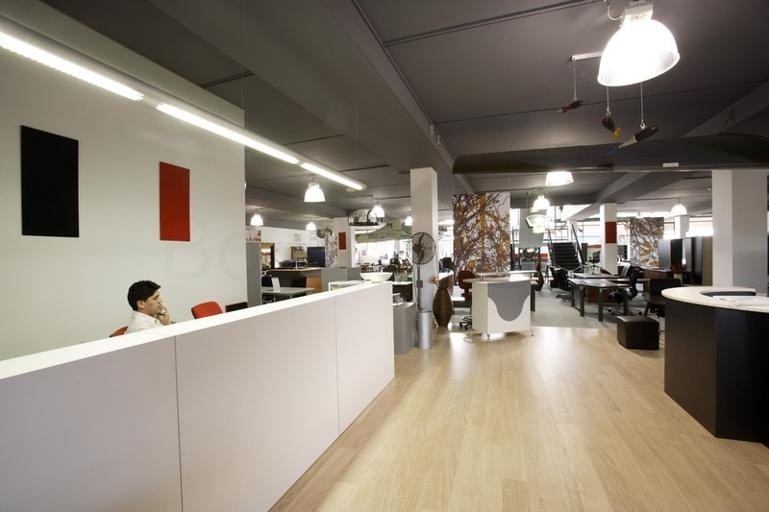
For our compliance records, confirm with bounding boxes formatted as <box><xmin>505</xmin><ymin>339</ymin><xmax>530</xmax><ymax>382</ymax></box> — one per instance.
<box><xmin>307</xmin><ymin>246</ymin><xmax>325</xmax><ymax>267</ymax></box>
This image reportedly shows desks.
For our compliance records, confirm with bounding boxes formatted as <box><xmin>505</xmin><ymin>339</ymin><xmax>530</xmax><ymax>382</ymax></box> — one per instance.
<box><xmin>570</xmin><ymin>262</ymin><xmax>681</xmax><ymax>322</ymax></box>
<box><xmin>262</xmin><ymin>287</ymin><xmax>315</xmax><ymax>302</ymax></box>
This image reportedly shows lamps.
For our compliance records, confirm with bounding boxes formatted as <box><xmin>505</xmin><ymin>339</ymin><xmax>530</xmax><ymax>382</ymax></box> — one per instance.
<box><xmin>597</xmin><ymin>4</ymin><xmax>680</xmax><ymax>87</ymax></box>
<box><xmin>669</xmin><ymin>198</ymin><xmax>688</xmax><ymax>217</ymax></box>
<box><xmin>532</xmin><ymin>188</ymin><xmax>550</xmax><ymax>210</ymax></box>
<box><xmin>245</xmin><ymin>170</ymin><xmax>413</xmax><ymax>231</ymax></box>
<box><xmin>546</xmin><ymin>172</ymin><xmax>574</xmax><ymax>187</ymax></box>
<box><xmin>0</xmin><ymin>14</ymin><xmax>367</xmax><ymax>192</ymax></box>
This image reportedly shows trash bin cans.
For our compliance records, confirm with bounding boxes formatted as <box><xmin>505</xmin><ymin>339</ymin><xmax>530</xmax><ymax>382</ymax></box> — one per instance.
<box><xmin>417</xmin><ymin>310</ymin><xmax>433</xmax><ymax>349</ymax></box>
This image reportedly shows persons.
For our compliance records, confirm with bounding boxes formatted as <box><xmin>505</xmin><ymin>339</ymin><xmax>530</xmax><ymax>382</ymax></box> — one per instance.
<box><xmin>123</xmin><ymin>280</ymin><xmax>175</xmax><ymax>334</ymax></box>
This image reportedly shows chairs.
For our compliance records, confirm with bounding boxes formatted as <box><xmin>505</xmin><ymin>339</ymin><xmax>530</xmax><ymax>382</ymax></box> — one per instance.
<box><xmin>110</xmin><ymin>326</ymin><xmax>128</xmax><ymax>337</ymax></box>
<box><xmin>191</xmin><ymin>301</ymin><xmax>223</xmax><ymax>319</ymax></box>
<box><xmin>357</xmin><ymin>263</ymin><xmax>411</xmax><ymax>281</ymax></box>
<box><xmin>439</xmin><ymin>257</ymin><xmax>574</xmax><ymax>340</ymax></box>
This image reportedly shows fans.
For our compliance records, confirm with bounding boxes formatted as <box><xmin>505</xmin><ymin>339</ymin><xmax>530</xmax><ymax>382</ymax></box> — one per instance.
<box><xmin>406</xmin><ymin>232</ymin><xmax>436</xmax><ymax>310</ymax></box>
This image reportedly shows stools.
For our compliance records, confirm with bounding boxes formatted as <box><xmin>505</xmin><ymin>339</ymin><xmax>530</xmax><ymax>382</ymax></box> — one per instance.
<box><xmin>617</xmin><ymin>315</ymin><xmax>660</xmax><ymax>350</ymax></box>
